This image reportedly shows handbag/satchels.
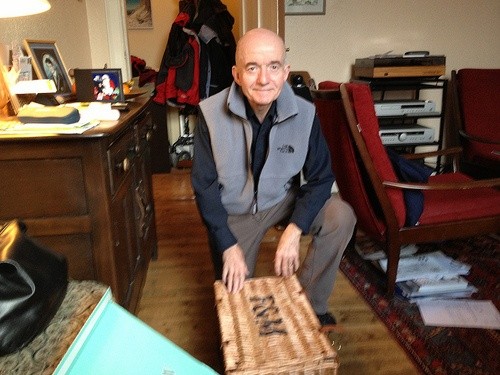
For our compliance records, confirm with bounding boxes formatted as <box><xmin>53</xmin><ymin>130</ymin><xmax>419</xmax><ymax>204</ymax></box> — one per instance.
<box><xmin>0</xmin><ymin>239</ymin><xmax>69</xmax><ymax>358</ymax></box>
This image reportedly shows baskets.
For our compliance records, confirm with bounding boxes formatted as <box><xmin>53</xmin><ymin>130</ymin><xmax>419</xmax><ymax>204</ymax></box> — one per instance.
<box><xmin>213</xmin><ymin>278</ymin><xmax>340</xmax><ymax>375</ymax></box>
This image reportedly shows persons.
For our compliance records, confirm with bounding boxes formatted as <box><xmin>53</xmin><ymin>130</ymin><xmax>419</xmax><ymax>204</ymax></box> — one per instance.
<box><xmin>45</xmin><ymin>58</ymin><xmax>116</xmax><ymax>103</ymax></box>
<box><xmin>191</xmin><ymin>28</ymin><xmax>356</xmax><ymax>332</ymax></box>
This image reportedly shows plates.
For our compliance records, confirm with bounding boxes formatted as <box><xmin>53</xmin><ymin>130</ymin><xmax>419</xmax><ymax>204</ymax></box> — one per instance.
<box><xmin>123</xmin><ymin>89</ymin><xmax>148</xmax><ymax>100</ymax></box>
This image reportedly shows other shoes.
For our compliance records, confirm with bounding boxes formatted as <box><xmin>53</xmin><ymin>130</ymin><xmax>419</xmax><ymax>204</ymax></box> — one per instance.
<box><xmin>317</xmin><ymin>312</ymin><xmax>336</xmax><ymax>333</ymax></box>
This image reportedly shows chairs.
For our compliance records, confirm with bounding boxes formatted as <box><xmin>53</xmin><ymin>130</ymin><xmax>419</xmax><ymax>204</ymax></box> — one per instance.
<box><xmin>308</xmin><ymin>68</ymin><xmax>500</xmax><ymax>301</ymax></box>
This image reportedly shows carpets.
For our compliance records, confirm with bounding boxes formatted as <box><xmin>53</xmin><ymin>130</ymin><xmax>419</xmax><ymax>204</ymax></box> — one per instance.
<box><xmin>339</xmin><ymin>232</ymin><xmax>500</xmax><ymax>375</ymax></box>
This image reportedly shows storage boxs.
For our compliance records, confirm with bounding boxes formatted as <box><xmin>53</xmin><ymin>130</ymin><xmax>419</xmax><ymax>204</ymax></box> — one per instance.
<box><xmin>214</xmin><ymin>273</ymin><xmax>341</xmax><ymax>375</ymax></box>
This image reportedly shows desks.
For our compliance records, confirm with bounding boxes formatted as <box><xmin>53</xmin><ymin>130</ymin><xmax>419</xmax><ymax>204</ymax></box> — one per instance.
<box><xmin>0</xmin><ymin>280</ymin><xmax>120</xmax><ymax>375</ymax></box>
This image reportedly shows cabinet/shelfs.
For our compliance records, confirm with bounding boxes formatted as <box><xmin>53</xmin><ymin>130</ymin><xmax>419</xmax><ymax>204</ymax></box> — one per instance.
<box><xmin>0</xmin><ymin>93</ymin><xmax>168</xmax><ymax>316</ymax></box>
<box><xmin>351</xmin><ymin>79</ymin><xmax>448</xmax><ymax>179</ymax></box>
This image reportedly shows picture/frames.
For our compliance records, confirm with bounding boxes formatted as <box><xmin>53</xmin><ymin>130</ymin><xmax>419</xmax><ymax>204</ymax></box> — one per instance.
<box><xmin>92</xmin><ymin>70</ymin><xmax>125</xmax><ymax>104</ymax></box>
<box><xmin>284</xmin><ymin>0</ymin><xmax>326</xmax><ymax>15</ymax></box>
<box><xmin>127</xmin><ymin>0</ymin><xmax>154</xmax><ymax>29</ymax></box>
<box><xmin>23</xmin><ymin>38</ymin><xmax>73</xmax><ymax>98</ymax></box>
